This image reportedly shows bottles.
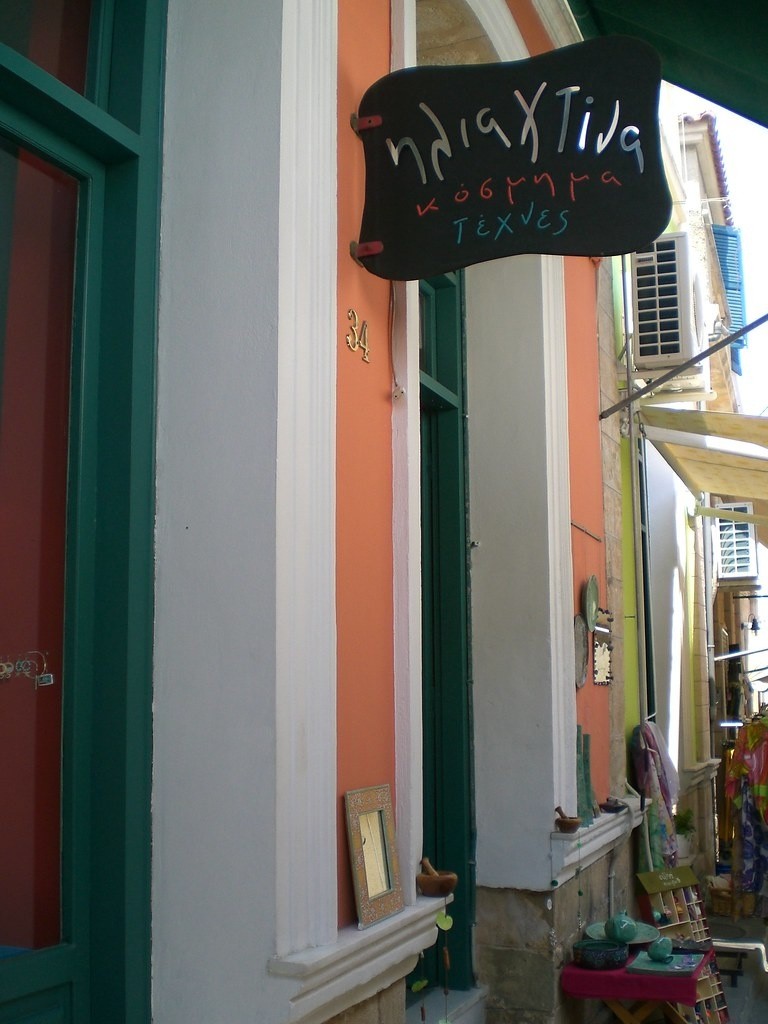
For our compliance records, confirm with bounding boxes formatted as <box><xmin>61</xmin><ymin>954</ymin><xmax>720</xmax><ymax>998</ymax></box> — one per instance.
<box><xmin>647</xmin><ymin>937</ymin><xmax>674</xmax><ymax>963</ymax></box>
<box><xmin>575</xmin><ymin>614</ymin><xmax>589</xmax><ymax>689</ymax></box>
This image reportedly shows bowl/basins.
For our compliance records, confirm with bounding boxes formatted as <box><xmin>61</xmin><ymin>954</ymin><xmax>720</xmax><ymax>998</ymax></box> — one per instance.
<box><xmin>573</xmin><ymin>939</ymin><xmax>629</xmax><ymax>970</ymax></box>
<box><xmin>416</xmin><ymin>870</ymin><xmax>457</xmax><ymax>897</ymax></box>
<box><xmin>555</xmin><ymin>815</ymin><xmax>582</xmax><ymax>832</ymax></box>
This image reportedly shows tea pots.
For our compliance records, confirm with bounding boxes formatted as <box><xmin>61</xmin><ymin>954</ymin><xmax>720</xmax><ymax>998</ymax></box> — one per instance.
<box><xmin>605</xmin><ymin>909</ymin><xmax>638</xmax><ymax>943</ymax></box>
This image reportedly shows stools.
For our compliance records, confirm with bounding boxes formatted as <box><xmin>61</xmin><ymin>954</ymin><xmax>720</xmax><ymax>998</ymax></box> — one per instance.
<box><xmin>679</xmin><ymin>936</ymin><xmax>762</xmax><ymax>988</ymax></box>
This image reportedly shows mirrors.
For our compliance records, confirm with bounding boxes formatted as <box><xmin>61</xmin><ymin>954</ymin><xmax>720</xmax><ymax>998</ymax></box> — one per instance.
<box><xmin>593</xmin><ymin>608</ymin><xmax>613</xmax><ymax>685</ymax></box>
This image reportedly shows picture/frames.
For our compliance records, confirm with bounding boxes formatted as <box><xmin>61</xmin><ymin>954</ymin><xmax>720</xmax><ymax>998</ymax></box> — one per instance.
<box><xmin>343</xmin><ymin>783</ymin><xmax>405</xmax><ymax>931</ymax></box>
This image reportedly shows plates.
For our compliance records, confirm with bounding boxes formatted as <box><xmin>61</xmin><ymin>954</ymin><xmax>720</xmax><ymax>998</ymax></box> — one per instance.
<box><xmin>585</xmin><ymin>918</ymin><xmax>660</xmax><ymax>945</ymax></box>
<box><xmin>581</xmin><ymin>575</ymin><xmax>599</xmax><ymax>632</ymax></box>
<box><xmin>626</xmin><ymin>950</ymin><xmax>704</xmax><ymax>976</ymax></box>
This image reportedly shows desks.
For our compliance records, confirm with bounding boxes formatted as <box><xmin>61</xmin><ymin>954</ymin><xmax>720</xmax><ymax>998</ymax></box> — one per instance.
<box><xmin>561</xmin><ymin>947</ymin><xmax>715</xmax><ymax>1024</ymax></box>
